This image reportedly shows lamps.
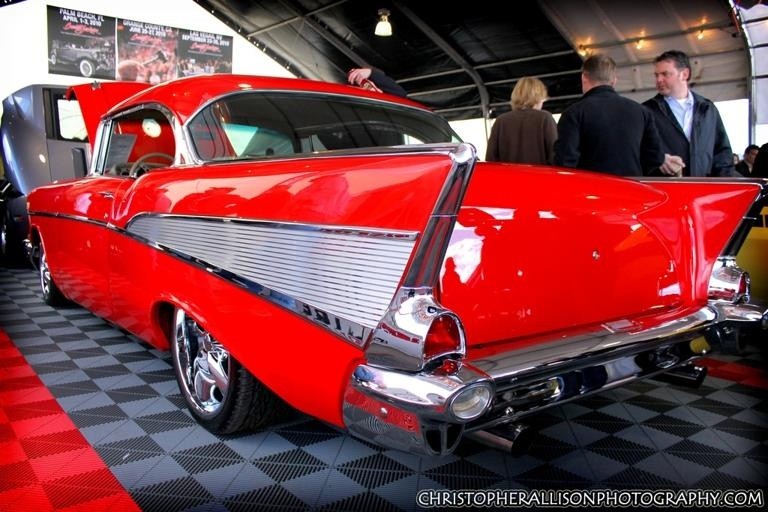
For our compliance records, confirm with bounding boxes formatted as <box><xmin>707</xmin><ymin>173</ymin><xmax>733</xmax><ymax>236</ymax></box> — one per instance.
<box><xmin>584</xmin><ymin>47</ymin><xmax>594</xmax><ymax>59</ymax></box>
<box><xmin>696</xmin><ymin>31</ymin><xmax>705</xmax><ymax>40</ymax></box>
<box><xmin>373</xmin><ymin>3</ymin><xmax>393</xmax><ymax>36</ymax></box>
<box><xmin>635</xmin><ymin>38</ymin><xmax>645</xmax><ymax>49</ymax></box>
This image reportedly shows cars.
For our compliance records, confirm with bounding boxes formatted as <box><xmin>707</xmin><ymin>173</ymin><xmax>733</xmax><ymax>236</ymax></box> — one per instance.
<box><xmin>50</xmin><ymin>42</ymin><xmax>113</xmax><ymax>77</ymax></box>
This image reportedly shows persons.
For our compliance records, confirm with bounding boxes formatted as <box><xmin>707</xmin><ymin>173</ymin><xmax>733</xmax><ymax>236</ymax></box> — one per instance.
<box><xmin>557</xmin><ymin>55</ymin><xmax>664</xmax><ymax>179</ymax></box>
<box><xmin>347</xmin><ymin>62</ymin><xmax>407</xmax><ymax>99</ymax></box>
<box><xmin>485</xmin><ymin>75</ymin><xmax>557</xmax><ymax>166</ymax></box>
<box><xmin>731</xmin><ymin>154</ymin><xmax>739</xmax><ymax>165</ymax></box>
<box><xmin>735</xmin><ymin>145</ymin><xmax>759</xmax><ymax>178</ymax></box>
<box><xmin>643</xmin><ymin>50</ymin><xmax>735</xmax><ymax>180</ymax></box>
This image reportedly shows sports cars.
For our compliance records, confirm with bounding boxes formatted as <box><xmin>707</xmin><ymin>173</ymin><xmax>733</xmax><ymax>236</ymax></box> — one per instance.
<box><xmin>25</xmin><ymin>74</ymin><xmax>758</xmax><ymax>461</ymax></box>
